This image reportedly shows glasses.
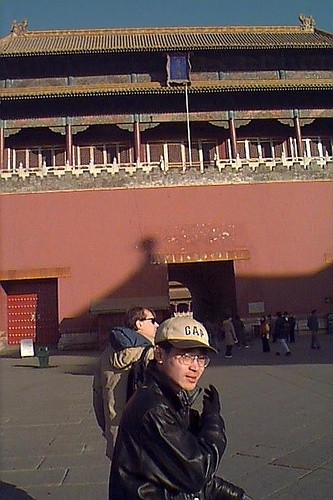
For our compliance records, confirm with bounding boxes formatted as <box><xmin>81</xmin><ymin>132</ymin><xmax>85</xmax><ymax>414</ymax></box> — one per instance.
<box><xmin>143</xmin><ymin>316</ymin><xmax>158</xmax><ymax>324</ymax></box>
<box><xmin>169</xmin><ymin>347</ymin><xmax>211</xmax><ymax>368</ymax></box>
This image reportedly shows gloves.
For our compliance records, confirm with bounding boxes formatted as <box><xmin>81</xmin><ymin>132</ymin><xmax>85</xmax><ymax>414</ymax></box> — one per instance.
<box><xmin>202</xmin><ymin>385</ymin><xmax>221</xmax><ymax>415</ymax></box>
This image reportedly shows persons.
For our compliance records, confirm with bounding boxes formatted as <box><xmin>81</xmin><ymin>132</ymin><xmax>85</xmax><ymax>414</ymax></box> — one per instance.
<box><xmin>221</xmin><ymin>317</ymin><xmax>250</xmax><ymax>358</ymax></box>
<box><xmin>307</xmin><ymin>310</ymin><xmax>322</xmax><ymax>349</ymax></box>
<box><xmin>259</xmin><ymin>311</ymin><xmax>299</xmax><ymax>357</ymax></box>
<box><xmin>92</xmin><ymin>306</ymin><xmax>160</xmax><ymax>462</ymax></box>
<box><xmin>108</xmin><ymin>318</ymin><xmax>254</xmax><ymax>500</ymax></box>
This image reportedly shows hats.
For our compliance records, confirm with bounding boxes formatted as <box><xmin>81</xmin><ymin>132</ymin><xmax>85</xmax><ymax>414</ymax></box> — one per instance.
<box><xmin>154</xmin><ymin>316</ymin><xmax>220</xmax><ymax>355</ymax></box>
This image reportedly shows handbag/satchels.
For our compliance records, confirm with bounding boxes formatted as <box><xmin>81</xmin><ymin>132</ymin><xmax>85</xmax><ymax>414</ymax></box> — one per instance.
<box><xmin>127</xmin><ymin>345</ymin><xmax>155</xmax><ymax>403</ymax></box>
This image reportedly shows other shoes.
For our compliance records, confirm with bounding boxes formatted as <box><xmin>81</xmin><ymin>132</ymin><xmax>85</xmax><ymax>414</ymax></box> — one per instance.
<box><xmin>286</xmin><ymin>351</ymin><xmax>292</xmax><ymax>355</ymax></box>
<box><xmin>225</xmin><ymin>355</ymin><xmax>233</xmax><ymax>357</ymax></box>
<box><xmin>276</xmin><ymin>352</ymin><xmax>281</xmax><ymax>355</ymax></box>
